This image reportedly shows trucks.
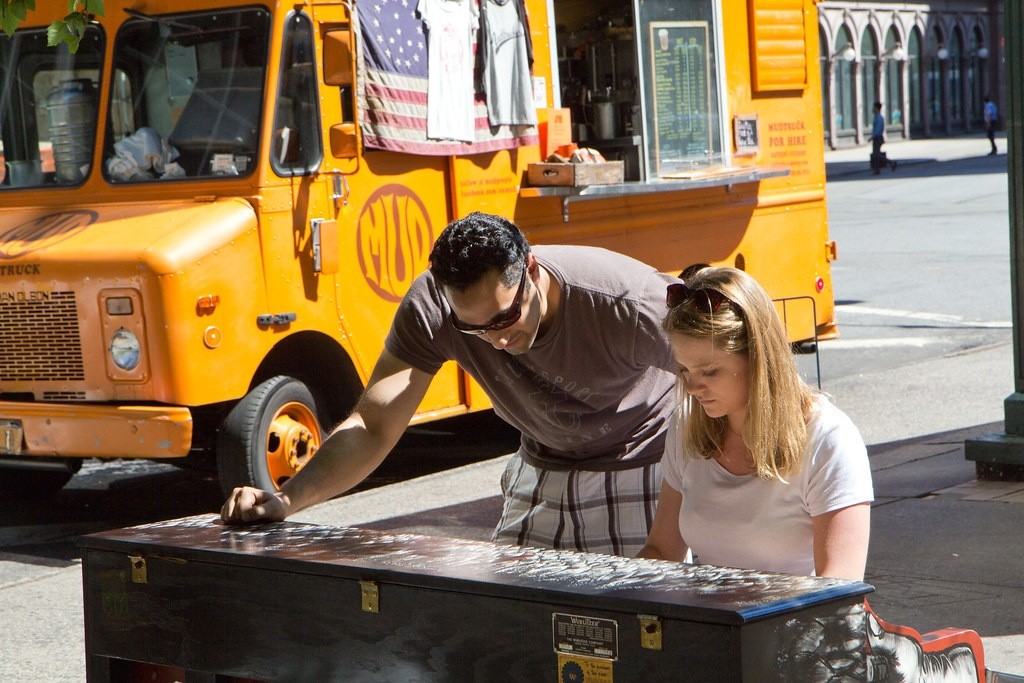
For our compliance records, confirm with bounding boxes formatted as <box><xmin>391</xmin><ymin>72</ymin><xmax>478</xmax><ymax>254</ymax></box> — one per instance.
<box><xmin>3</xmin><ymin>0</ymin><xmax>836</xmax><ymax>512</ymax></box>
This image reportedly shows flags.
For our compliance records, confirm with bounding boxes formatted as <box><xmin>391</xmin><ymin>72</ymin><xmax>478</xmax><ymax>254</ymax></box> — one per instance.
<box><xmin>356</xmin><ymin>0</ymin><xmax>540</xmax><ymax>157</ymax></box>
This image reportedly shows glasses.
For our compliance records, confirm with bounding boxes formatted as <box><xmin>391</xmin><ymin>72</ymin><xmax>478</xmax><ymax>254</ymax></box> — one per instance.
<box><xmin>450</xmin><ymin>263</ymin><xmax>527</xmax><ymax>336</ymax></box>
<box><xmin>665</xmin><ymin>283</ymin><xmax>746</xmax><ymax>320</ymax></box>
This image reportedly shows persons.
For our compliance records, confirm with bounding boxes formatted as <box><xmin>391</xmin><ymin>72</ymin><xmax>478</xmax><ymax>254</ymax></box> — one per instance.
<box><xmin>982</xmin><ymin>99</ymin><xmax>999</xmax><ymax>157</ymax></box>
<box><xmin>632</xmin><ymin>260</ymin><xmax>872</xmax><ymax>584</ymax></box>
<box><xmin>870</xmin><ymin>100</ymin><xmax>898</xmax><ymax>173</ymax></box>
<box><xmin>220</xmin><ymin>212</ymin><xmax>703</xmax><ymax>565</ymax></box>
